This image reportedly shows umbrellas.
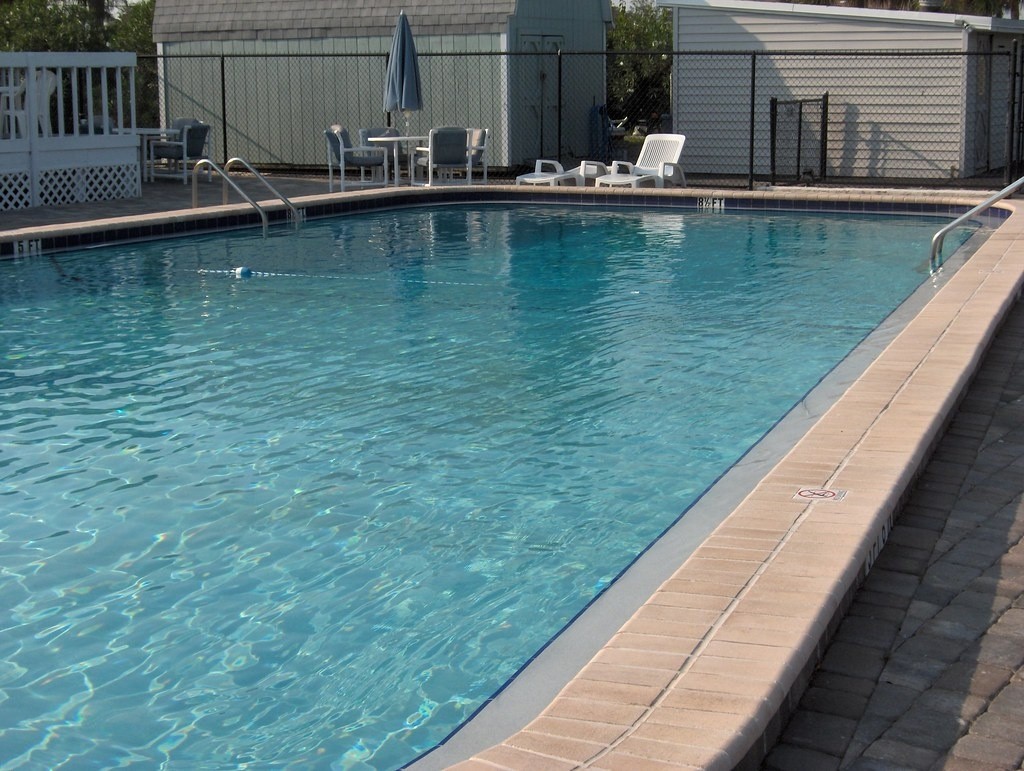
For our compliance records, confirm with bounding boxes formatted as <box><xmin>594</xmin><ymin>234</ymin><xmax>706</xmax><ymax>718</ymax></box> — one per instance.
<box><xmin>382</xmin><ymin>9</ymin><xmax>423</xmax><ymax>181</ymax></box>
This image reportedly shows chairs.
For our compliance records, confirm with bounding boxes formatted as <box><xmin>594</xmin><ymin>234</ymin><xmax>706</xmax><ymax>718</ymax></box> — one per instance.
<box><xmin>416</xmin><ymin>127</ymin><xmax>473</xmax><ymax>187</ymax></box>
<box><xmin>443</xmin><ymin>128</ymin><xmax>488</xmax><ymax>185</ymax></box>
<box><xmin>85</xmin><ymin>116</ymin><xmax>213</xmax><ymax>185</ymax></box>
<box><xmin>323</xmin><ymin>125</ymin><xmax>388</xmax><ymax>192</ymax></box>
<box><xmin>516</xmin><ymin>160</ymin><xmax>620</xmax><ymax>187</ymax></box>
<box><xmin>359</xmin><ymin>127</ymin><xmax>417</xmax><ymax>183</ymax></box>
<box><xmin>594</xmin><ymin>134</ymin><xmax>687</xmax><ymax>188</ymax></box>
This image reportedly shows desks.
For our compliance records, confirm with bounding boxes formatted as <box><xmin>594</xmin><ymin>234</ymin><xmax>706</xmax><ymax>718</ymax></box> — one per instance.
<box><xmin>112</xmin><ymin>127</ymin><xmax>181</xmax><ymax>183</ymax></box>
<box><xmin>367</xmin><ymin>135</ymin><xmax>429</xmax><ymax>187</ymax></box>
